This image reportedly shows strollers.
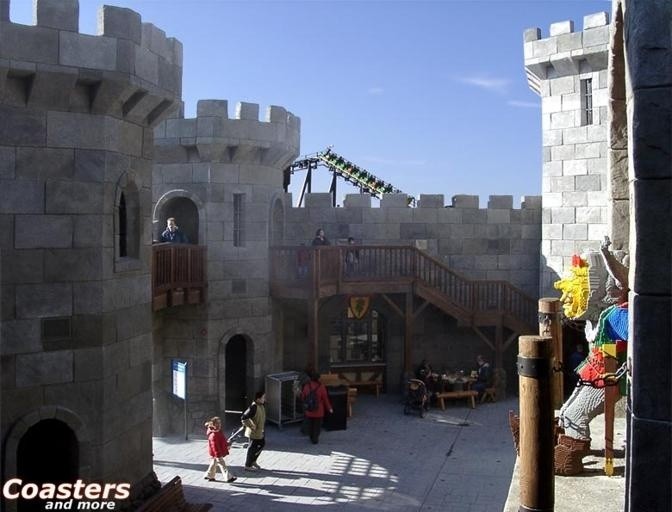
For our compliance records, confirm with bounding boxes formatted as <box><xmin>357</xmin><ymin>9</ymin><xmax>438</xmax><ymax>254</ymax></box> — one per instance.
<box><xmin>403</xmin><ymin>379</ymin><xmax>429</xmax><ymax>418</ymax></box>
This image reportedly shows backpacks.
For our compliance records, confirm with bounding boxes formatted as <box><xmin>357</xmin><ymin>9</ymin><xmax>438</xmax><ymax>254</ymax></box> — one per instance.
<box><xmin>301</xmin><ymin>383</ymin><xmax>321</xmax><ymax>412</ymax></box>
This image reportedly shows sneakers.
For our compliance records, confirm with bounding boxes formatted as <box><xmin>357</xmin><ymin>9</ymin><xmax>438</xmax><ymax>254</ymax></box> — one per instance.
<box><xmin>204</xmin><ymin>462</ymin><xmax>260</xmax><ymax>483</ymax></box>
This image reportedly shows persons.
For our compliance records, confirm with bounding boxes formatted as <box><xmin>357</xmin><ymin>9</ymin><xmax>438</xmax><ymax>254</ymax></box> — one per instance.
<box><xmin>420</xmin><ymin>358</ymin><xmax>431</xmax><ymax>374</ymax></box>
<box><xmin>301</xmin><ymin>371</ymin><xmax>334</xmax><ymax>445</ymax></box>
<box><xmin>312</xmin><ymin>228</ymin><xmax>331</xmax><ymax>246</ymax></box>
<box><xmin>203</xmin><ymin>416</ymin><xmax>237</xmax><ymax>484</ymax></box>
<box><xmin>472</xmin><ymin>354</ymin><xmax>493</xmax><ymax>404</ymax></box>
<box><xmin>240</xmin><ymin>392</ymin><xmax>267</xmax><ymax>471</ymax></box>
<box><xmin>419</xmin><ymin>368</ymin><xmax>438</xmax><ymax>395</ymax></box>
<box><xmin>159</xmin><ymin>217</ymin><xmax>192</xmax><ymax>244</ymax></box>
<box><xmin>344</xmin><ymin>236</ymin><xmax>359</xmax><ymax>274</ymax></box>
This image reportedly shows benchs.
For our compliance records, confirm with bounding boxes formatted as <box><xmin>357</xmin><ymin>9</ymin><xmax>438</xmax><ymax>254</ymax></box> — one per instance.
<box><xmin>134</xmin><ymin>476</ymin><xmax>213</xmax><ymax>512</ymax></box>
<box><xmin>481</xmin><ymin>387</ymin><xmax>497</xmax><ymax>403</ymax></box>
<box><xmin>348</xmin><ymin>380</ymin><xmax>384</xmax><ymax>400</ymax></box>
<box><xmin>436</xmin><ymin>390</ymin><xmax>479</xmax><ymax>411</ymax></box>
